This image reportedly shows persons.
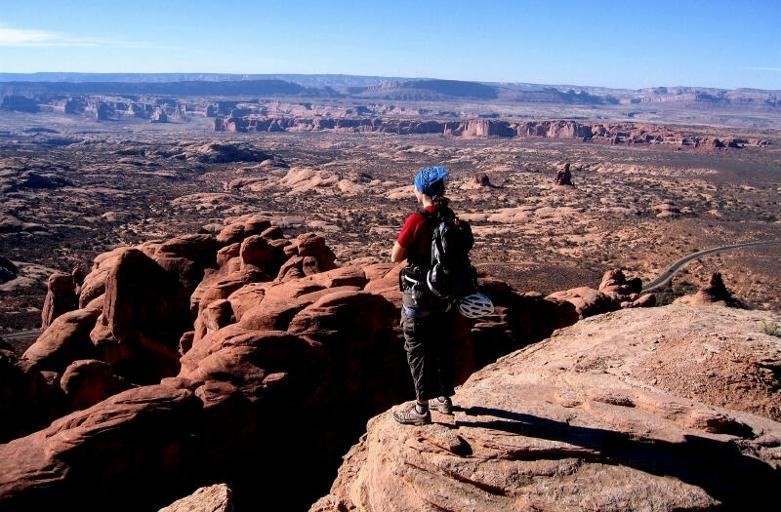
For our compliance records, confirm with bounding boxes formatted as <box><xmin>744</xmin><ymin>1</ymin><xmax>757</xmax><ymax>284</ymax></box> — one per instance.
<box><xmin>391</xmin><ymin>166</ymin><xmax>474</xmax><ymax>425</ymax></box>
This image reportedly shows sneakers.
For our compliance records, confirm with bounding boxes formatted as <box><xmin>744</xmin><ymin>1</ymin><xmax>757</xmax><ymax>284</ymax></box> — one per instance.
<box><xmin>392</xmin><ymin>406</ymin><xmax>431</xmax><ymax>426</ymax></box>
<box><xmin>428</xmin><ymin>397</ymin><xmax>452</xmax><ymax>414</ymax></box>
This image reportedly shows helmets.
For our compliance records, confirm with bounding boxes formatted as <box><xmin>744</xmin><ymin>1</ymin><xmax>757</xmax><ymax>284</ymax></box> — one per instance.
<box><xmin>457</xmin><ymin>292</ymin><xmax>495</xmax><ymax>320</ymax></box>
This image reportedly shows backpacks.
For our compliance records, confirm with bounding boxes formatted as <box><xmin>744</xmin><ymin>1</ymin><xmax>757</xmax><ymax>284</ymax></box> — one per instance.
<box><xmin>402</xmin><ymin>209</ymin><xmax>473</xmax><ymax>300</ymax></box>
